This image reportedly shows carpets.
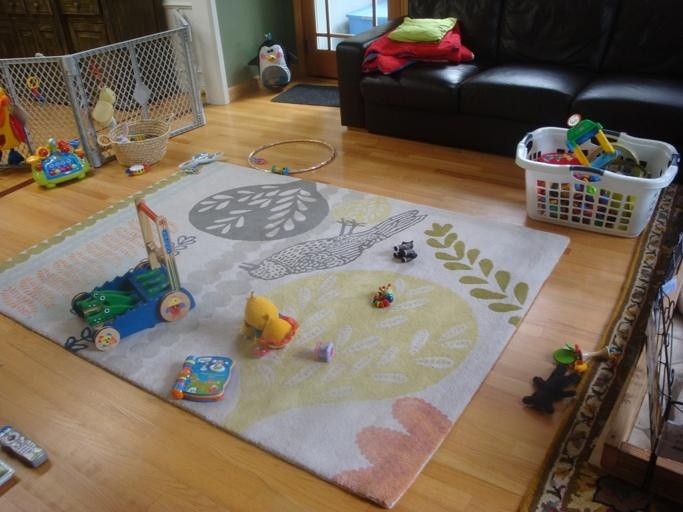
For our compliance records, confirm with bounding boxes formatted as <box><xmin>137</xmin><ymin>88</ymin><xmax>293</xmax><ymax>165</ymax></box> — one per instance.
<box><xmin>268</xmin><ymin>79</ymin><xmax>345</xmax><ymax>115</ymax></box>
<box><xmin>0</xmin><ymin>154</ymin><xmax>573</xmax><ymax>512</ymax></box>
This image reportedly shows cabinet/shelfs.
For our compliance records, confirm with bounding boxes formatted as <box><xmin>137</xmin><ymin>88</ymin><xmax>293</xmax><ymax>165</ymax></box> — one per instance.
<box><xmin>0</xmin><ymin>0</ymin><xmax>180</xmax><ymax>115</ymax></box>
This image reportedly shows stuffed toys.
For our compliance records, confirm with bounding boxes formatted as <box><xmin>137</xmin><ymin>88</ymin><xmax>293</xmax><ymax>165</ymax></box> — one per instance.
<box><xmin>393</xmin><ymin>239</ymin><xmax>416</xmax><ymax>263</ymax></box>
<box><xmin>248</xmin><ymin>31</ymin><xmax>297</xmax><ymax>91</ymax></box>
<box><xmin>523</xmin><ymin>363</ymin><xmax>579</xmax><ymax>414</ymax></box>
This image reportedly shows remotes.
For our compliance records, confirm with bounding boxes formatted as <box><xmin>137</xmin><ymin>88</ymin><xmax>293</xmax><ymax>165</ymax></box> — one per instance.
<box><xmin>0</xmin><ymin>425</ymin><xmax>48</xmax><ymax>468</ymax></box>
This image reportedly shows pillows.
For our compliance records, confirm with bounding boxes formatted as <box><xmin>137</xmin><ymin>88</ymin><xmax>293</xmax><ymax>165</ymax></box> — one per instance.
<box><xmin>384</xmin><ymin>11</ymin><xmax>460</xmax><ymax>50</ymax></box>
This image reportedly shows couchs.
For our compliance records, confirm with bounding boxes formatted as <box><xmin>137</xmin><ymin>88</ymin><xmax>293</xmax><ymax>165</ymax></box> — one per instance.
<box><xmin>329</xmin><ymin>0</ymin><xmax>681</xmax><ymax>189</ymax></box>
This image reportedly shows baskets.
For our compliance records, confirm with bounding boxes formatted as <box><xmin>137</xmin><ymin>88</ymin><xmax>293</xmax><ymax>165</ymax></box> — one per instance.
<box><xmin>109</xmin><ymin>118</ymin><xmax>171</xmax><ymax>167</ymax></box>
<box><xmin>514</xmin><ymin>124</ymin><xmax>680</xmax><ymax>239</ymax></box>
